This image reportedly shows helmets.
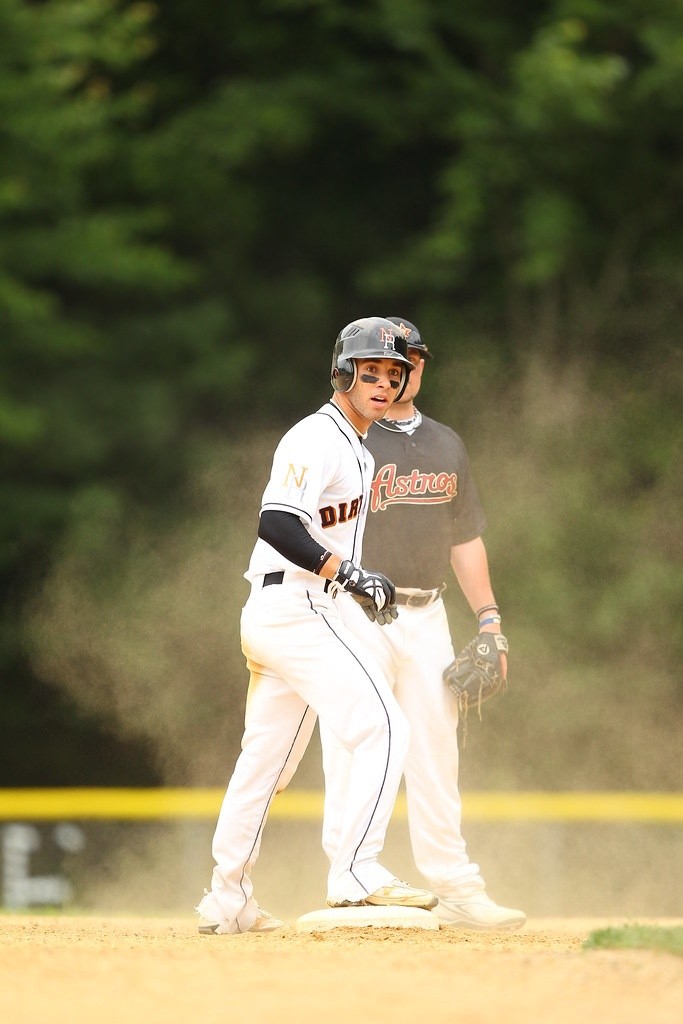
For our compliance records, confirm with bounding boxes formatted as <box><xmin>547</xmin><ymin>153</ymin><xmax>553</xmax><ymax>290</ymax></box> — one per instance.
<box><xmin>385</xmin><ymin>316</ymin><xmax>434</xmax><ymax>359</ymax></box>
<box><xmin>330</xmin><ymin>316</ymin><xmax>417</xmax><ymax>403</ymax></box>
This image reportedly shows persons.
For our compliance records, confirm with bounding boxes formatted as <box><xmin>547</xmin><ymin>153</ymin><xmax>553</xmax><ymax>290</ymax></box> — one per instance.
<box><xmin>320</xmin><ymin>316</ymin><xmax>527</xmax><ymax>933</ymax></box>
<box><xmin>195</xmin><ymin>317</ymin><xmax>438</xmax><ymax>934</ymax></box>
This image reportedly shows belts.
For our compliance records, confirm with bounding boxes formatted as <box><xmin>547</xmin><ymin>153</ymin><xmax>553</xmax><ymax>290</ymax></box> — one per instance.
<box><xmin>395</xmin><ymin>583</ymin><xmax>446</xmax><ymax>608</ymax></box>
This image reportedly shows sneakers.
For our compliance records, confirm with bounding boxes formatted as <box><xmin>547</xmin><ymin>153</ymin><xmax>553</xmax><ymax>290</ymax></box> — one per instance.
<box><xmin>430</xmin><ymin>883</ymin><xmax>527</xmax><ymax>932</ymax></box>
<box><xmin>197</xmin><ymin>893</ymin><xmax>286</xmax><ymax>934</ymax></box>
<box><xmin>325</xmin><ymin>864</ymin><xmax>440</xmax><ymax>909</ymax></box>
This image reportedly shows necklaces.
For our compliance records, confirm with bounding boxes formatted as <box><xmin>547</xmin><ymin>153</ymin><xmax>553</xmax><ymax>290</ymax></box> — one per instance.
<box><xmin>328</xmin><ymin>398</ymin><xmax>369</xmax><ymax>440</ymax></box>
<box><xmin>371</xmin><ymin>405</ymin><xmax>423</xmax><ymax>433</ymax></box>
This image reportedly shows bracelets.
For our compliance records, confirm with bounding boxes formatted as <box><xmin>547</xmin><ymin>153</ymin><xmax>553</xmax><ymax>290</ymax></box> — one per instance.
<box><xmin>479</xmin><ymin>615</ymin><xmax>501</xmax><ymax>628</ymax></box>
<box><xmin>475</xmin><ymin>604</ymin><xmax>499</xmax><ymax>621</ymax></box>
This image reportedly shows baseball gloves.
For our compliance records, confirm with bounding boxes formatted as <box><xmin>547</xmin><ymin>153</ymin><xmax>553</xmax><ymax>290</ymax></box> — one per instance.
<box><xmin>443</xmin><ymin>630</ymin><xmax>508</xmax><ymax>747</ymax></box>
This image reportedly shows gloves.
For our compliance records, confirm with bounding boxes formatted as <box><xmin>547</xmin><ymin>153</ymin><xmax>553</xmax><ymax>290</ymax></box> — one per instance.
<box><xmin>327</xmin><ymin>560</ymin><xmax>399</xmax><ymax>626</ymax></box>
